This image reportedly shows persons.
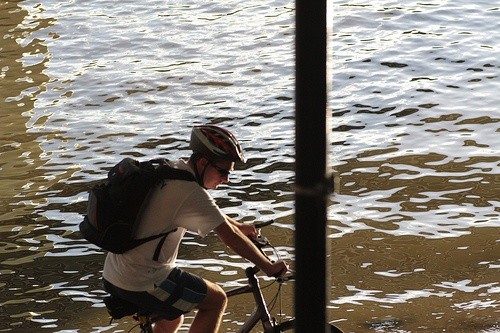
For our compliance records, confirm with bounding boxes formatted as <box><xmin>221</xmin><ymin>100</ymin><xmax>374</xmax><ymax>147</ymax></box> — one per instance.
<box><xmin>102</xmin><ymin>125</ymin><xmax>288</xmax><ymax>333</ymax></box>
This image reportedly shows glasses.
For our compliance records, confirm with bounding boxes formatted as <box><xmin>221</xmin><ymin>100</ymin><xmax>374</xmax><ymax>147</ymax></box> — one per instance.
<box><xmin>206</xmin><ymin>156</ymin><xmax>234</xmax><ymax>177</ymax></box>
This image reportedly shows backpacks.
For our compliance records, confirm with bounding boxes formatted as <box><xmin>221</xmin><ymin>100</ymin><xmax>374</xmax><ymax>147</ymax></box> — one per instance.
<box><xmin>79</xmin><ymin>156</ymin><xmax>201</xmax><ymax>262</ymax></box>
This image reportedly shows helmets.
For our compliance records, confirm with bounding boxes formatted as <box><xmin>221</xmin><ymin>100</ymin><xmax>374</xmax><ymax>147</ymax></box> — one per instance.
<box><xmin>190</xmin><ymin>124</ymin><xmax>247</xmax><ymax>165</ymax></box>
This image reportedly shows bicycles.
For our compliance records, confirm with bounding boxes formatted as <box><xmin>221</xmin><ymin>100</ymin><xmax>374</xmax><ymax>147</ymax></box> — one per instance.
<box><xmin>103</xmin><ymin>220</ymin><xmax>343</xmax><ymax>333</ymax></box>
